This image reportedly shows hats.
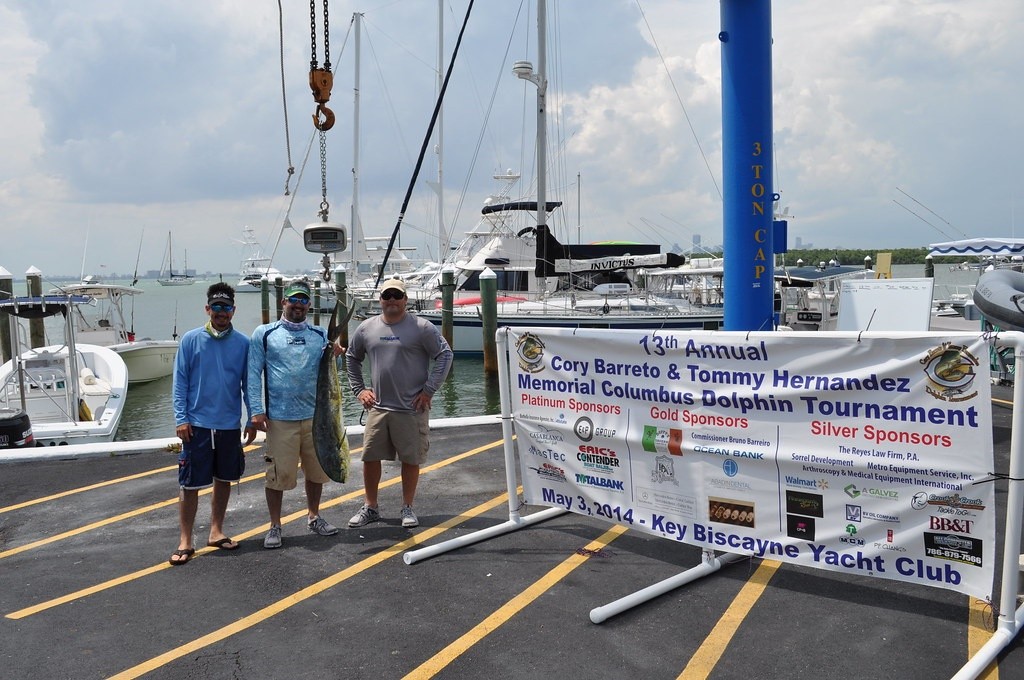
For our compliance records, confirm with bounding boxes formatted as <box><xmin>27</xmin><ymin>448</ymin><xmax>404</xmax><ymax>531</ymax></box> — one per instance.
<box><xmin>208</xmin><ymin>291</ymin><xmax>234</xmax><ymax>306</ymax></box>
<box><xmin>379</xmin><ymin>279</ymin><xmax>407</xmax><ymax>299</ymax></box>
<box><xmin>284</xmin><ymin>283</ymin><xmax>311</xmax><ymax>300</ymax></box>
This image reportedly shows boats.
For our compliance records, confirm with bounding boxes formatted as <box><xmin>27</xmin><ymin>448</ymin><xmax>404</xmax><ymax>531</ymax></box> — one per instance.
<box><xmin>232</xmin><ymin>224</ymin><xmax>289</xmax><ymax>293</ymax></box>
<box><xmin>52</xmin><ymin>280</ymin><xmax>182</xmax><ymax>384</ymax></box>
<box><xmin>408</xmin><ymin>3</ymin><xmax>733</xmax><ymax>360</ymax></box>
<box><xmin>0</xmin><ymin>294</ymin><xmax>128</xmax><ymax>449</ymax></box>
<box><xmin>156</xmin><ymin>229</ymin><xmax>197</xmax><ymax>287</ymax></box>
<box><xmin>925</xmin><ymin>235</ymin><xmax>1024</xmax><ymax>331</ymax></box>
<box><xmin>770</xmin><ymin>262</ymin><xmax>871</xmax><ymax>319</ymax></box>
<box><xmin>279</xmin><ymin>11</ymin><xmax>430</xmax><ymax>313</ymax></box>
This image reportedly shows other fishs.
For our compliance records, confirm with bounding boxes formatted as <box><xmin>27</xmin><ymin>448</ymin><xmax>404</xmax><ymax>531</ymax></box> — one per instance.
<box><xmin>311</xmin><ymin>300</ymin><xmax>356</xmax><ymax>484</ymax></box>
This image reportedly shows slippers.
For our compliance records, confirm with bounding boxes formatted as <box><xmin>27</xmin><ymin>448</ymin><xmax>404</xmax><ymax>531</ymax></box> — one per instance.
<box><xmin>206</xmin><ymin>537</ymin><xmax>240</xmax><ymax>550</ymax></box>
<box><xmin>169</xmin><ymin>546</ymin><xmax>194</xmax><ymax>565</ymax></box>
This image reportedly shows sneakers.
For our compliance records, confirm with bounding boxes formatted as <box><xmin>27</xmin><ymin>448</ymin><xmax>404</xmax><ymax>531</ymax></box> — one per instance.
<box><xmin>264</xmin><ymin>524</ymin><xmax>282</xmax><ymax>547</ymax></box>
<box><xmin>401</xmin><ymin>506</ymin><xmax>419</xmax><ymax>527</ymax></box>
<box><xmin>348</xmin><ymin>503</ymin><xmax>380</xmax><ymax>527</ymax></box>
<box><xmin>307</xmin><ymin>513</ymin><xmax>338</xmax><ymax>535</ymax></box>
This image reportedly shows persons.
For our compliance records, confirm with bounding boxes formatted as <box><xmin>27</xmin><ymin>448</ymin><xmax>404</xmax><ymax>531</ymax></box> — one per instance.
<box><xmin>345</xmin><ymin>278</ymin><xmax>455</xmax><ymax>528</ymax></box>
<box><xmin>167</xmin><ymin>281</ymin><xmax>259</xmax><ymax>566</ymax></box>
<box><xmin>247</xmin><ymin>282</ymin><xmax>345</xmax><ymax>550</ymax></box>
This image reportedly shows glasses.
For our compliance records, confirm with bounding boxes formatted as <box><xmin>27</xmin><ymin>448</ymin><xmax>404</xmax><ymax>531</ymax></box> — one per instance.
<box><xmin>288</xmin><ymin>296</ymin><xmax>309</xmax><ymax>305</ymax></box>
<box><xmin>211</xmin><ymin>305</ymin><xmax>233</xmax><ymax>310</ymax></box>
<box><xmin>382</xmin><ymin>291</ymin><xmax>405</xmax><ymax>299</ymax></box>
